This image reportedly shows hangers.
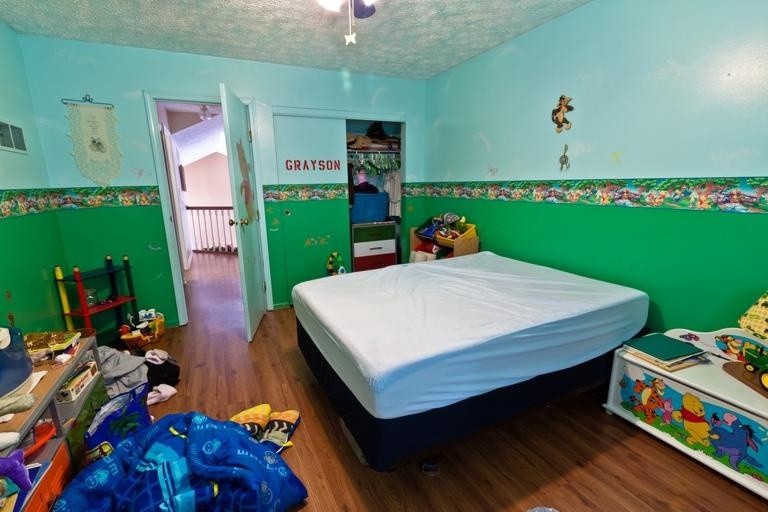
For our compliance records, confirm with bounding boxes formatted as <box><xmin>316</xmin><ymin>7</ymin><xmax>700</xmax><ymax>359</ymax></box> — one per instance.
<box><xmin>350</xmin><ymin>149</ymin><xmax>401</xmax><ymax>178</ymax></box>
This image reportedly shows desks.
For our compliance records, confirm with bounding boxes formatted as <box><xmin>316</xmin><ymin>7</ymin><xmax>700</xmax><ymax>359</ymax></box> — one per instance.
<box><xmin>0</xmin><ymin>336</ymin><xmax>105</xmax><ymax>511</ymax></box>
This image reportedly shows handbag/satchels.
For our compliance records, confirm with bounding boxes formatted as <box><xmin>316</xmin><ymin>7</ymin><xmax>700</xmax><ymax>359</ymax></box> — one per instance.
<box><xmin>84</xmin><ymin>382</ymin><xmax>153</xmax><ymax>461</ymax></box>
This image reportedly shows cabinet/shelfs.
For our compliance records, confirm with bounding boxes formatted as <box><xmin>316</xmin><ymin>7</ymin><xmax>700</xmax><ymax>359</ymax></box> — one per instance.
<box><xmin>51</xmin><ymin>252</ymin><xmax>138</xmax><ymax>354</ymax></box>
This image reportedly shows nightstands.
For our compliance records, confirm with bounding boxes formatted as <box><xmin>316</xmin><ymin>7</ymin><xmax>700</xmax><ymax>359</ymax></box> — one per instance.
<box><xmin>601</xmin><ymin>325</ymin><xmax>768</xmax><ymax>503</ymax></box>
<box><xmin>408</xmin><ymin>224</ymin><xmax>481</xmax><ymax>263</ymax></box>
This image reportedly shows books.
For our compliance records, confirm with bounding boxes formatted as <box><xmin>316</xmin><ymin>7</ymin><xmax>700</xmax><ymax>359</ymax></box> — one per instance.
<box><xmin>622</xmin><ymin>333</ymin><xmax>711</xmax><ymax>371</ymax></box>
<box><xmin>28</xmin><ymin>332</ymin><xmax>81</xmax><ymax>353</ymax></box>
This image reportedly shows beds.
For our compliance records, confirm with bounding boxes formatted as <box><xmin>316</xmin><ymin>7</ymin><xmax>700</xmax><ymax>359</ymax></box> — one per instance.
<box><xmin>291</xmin><ymin>247</ymin><xmax>650</xmax><ymax>473</ymax></box>
